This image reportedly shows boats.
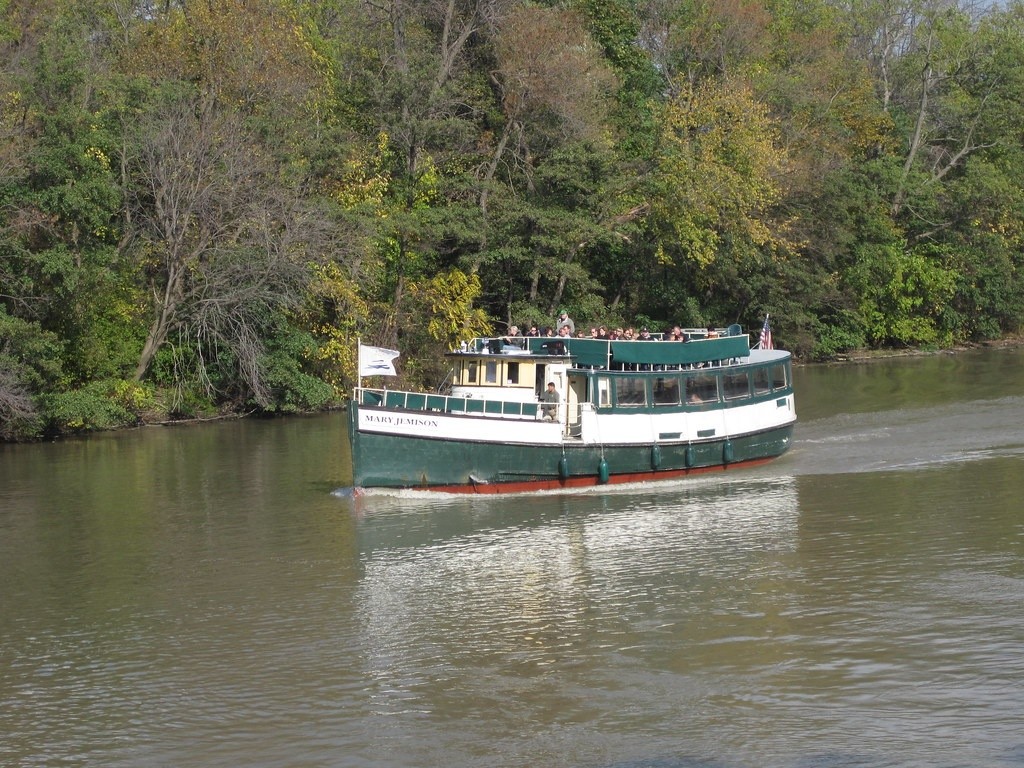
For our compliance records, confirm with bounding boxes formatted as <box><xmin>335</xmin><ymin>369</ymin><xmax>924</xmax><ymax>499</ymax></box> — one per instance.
<box><xmin>345</xmin><ymin>323</ymin><xmax>798</xmax><ymax>494</ymax></box>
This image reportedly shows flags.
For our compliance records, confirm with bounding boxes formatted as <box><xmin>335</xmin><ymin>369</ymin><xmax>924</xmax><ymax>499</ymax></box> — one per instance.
<box><xmin>760</xmin><ymin>317</ymin><xmax>773</xmax><ymax>350</ymax></box>
<box><xmin>360</xmin><ymin>344</ymin><xmax>400</xmax><ymax>376</ymax></box>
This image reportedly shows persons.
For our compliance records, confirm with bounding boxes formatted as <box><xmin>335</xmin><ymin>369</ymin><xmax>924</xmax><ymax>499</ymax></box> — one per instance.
<box><xmin>667</xmin><ymin>334</ymin><xmax>678</xmax><ymax>341</ymax></box>
<box><xmin>690</xmin><ymin>392</ymin><xmax>703</xmax><ymax>405</ymax></box>
<box><xmin>554</xmin><ymin>328</ymin><xmax>572</xmax><ymax>338</ymax></box>
<box><xmin>503</xmin><ymin>325</ymin><xmax>524</xmax><ymax>350</ymax></box>
<box><xmin>504</xmin><ymin>328</ymin><xmax>512</xmax><ymax>345</ymax></box>
<box><xmin>674</xmin><ymin>325</ymin><xmax>687</xmax><ymax>341</ymax></box>
<box><xmin>663</xmin><ymin>327</ymin><xmax>673</xmax><ymax>340</ymax></box>
<box><xmin>698</xmin><ymin>328</ymin><xmax>719</xmax><ymax>368</ymax></box>
<box><xmin>521</xmin><ymin>321</ymin><xmax>533</xmax><ymax>348</ymax></box>
<box><xmin>576</xmin><ymin>330</ymin><xmax>586</xmax><ymax>338</ymax></box>
<box><xmin>540</xmin><ymin>382</ymin><xmax>559</xmax><ymax>419</ymax></box>
<box><xmin>563</xmin><ymin>325</ymin><xmax>575</xmax><ymax>337</ymax></box>
<box><xmin>526</xmin><ymin>326</ymin><xmax>539</xmax><ymax>337</ymax></box>
<box><xmin>588</xmin><ymin>324</ymin><xmax>662</xmax><ymax>371</ymax></box>
<box><xmin>557</xmin><ymin>310</ymin><xmax>575</xmax><ymax>334</ymax></box>
<box><xmin>676</xmin><ymin>334</ymin><xmax>684</xmax><ymax>341</ymax></box>
<box><xmin>541</xmin><ymin>327</ymin><xmax>553</xmax><ymax>337</ymax></box>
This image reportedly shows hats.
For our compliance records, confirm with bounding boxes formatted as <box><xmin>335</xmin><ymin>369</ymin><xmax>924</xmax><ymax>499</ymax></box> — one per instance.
<box><xmin>559</xmin><ymin>310</ymin><xmax>568</xmax><ymax>315</ymax></box>
<box><xmin>639</xmin><ymin>326</ymin><xmax>649</xmax><ymax>332</ymax></box>
